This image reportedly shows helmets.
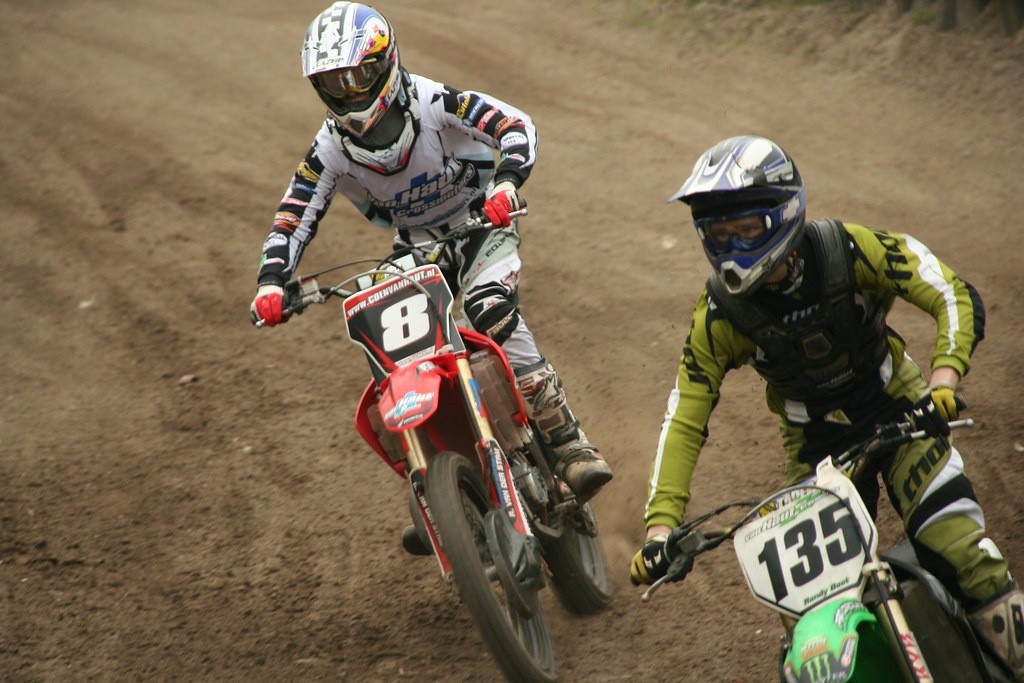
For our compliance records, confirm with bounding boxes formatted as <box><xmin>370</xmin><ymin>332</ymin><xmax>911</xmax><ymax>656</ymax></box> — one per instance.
<box><xmin>667</xmin><ymin>135</ymin><xmax>806</xmax><ymax>298</ymax></box>
<box><xmin>302</xmin><ymin>1</ymin><xmax>401</xmax><ymax>139</ymax></box>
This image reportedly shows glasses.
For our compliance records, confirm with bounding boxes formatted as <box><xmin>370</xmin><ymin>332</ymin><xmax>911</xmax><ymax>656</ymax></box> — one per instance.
<box><xmin>313</xmin><ymin>61</ymin><xmax>382</xmax><ymax>99</ymax></box>
<box><xmin>694</xmin><ymin>205</ymin><xmax>779</xmax><ymax>256</ymax></box>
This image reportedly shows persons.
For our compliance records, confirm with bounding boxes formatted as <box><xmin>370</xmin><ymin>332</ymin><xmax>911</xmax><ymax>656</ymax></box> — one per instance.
<box><xmin>247</xmin><ymin>0</ymin><xmax>615</xmax><ymax>557</ymax></box>
<box><xmin>628</xmin><ymin>133</ymin><xmax>1024</xmax><ymax>683</ymax></box>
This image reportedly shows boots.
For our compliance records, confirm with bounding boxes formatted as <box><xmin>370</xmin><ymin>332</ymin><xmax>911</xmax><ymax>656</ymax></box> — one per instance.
<box><xmin>399</xmin><ymin>524</ymin><xmax>432</xmax><ymax>555</ymax></box>
<box><xmin>966</xmin><ymin>589</ymin><xmax>1024</xmax><ymax>683</ymax></box>
<box><xmin>515</xmin><ymin>358</ymin><xmax>613</xmax><ymax>487</ymax></box>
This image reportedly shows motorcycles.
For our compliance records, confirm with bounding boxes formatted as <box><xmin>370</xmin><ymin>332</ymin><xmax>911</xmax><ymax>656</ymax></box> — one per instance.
<box><xmin>628</xmin><ymin>392</ymin><xmax>1024</xmax><ymax>683</ymax></box>
<box><xmin>247</xmin><ymin>193</ymin><xmax>614</xmax><ymax>683</ymax></box>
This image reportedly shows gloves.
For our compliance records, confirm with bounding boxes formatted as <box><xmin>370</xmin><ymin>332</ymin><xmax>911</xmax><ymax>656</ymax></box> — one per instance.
<box><xmin>629</xmin><ymin>533</ymin><xmax>678</xmax><ymax>586</ymax></box>
<box><xmin>484</xmin><ymin>180</ymin><xmax>519</xmax><ymax>227</ymax></box>
<box><xmin>908</xmin><ymin>382</ymin><xmax>961</xmax><ymax>441</ymax></box>
<box><xmin>250</xmin><ymin>285</ymin><xmax>289</xmax><ymax>328</ymax></box>
